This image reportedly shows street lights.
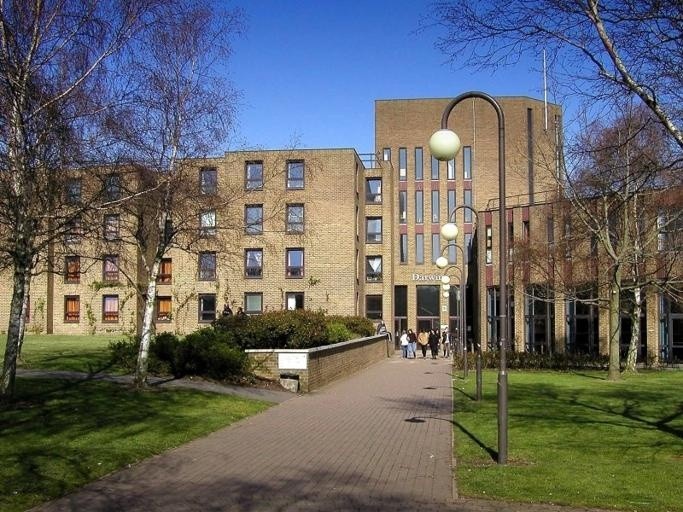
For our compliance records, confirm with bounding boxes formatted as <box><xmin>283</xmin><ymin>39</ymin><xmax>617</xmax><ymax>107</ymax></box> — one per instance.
<box><xmin>428</xmin><ymin>90</ymin><xmax>506</xmax><ymax>464</ymax></box>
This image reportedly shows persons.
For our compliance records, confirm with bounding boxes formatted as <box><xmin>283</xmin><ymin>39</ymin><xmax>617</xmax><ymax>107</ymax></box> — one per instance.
<box><xmin>376</xmin><ymin>321</ymin><xmax>392</xmax><ymax>342</ymax></box>
<box><xmin>222</xmin><ymin>304</ymin><xmax>233</xmax><ymax>317</ymax></box>
<box><xmin>400</xmin><ymin>329</ymin><xmax>410</xmax><ymax>359</ymax></box>
<box><xmin>407</xmin><ymin>329</ymin><xmax>417</xmax><ymax>359</ymax></box>
<box><xmin>417</xmin><ymin>329</ymin><xmax>429</xmax><ymax>359</ymax></box>
<box><xmin>236</xmin><ymin>307</ymin><xmax>245</xmax><ymax>315</ymax></box>
<box><xmin>442</xmin><ymin>327</ymin><xmax>451</xmax><ymax>357</ymax></box>
<box><xmin>427</xmin><ymin>329</ymin><xmax>441</xmax><ymax>359</ymax></box>
